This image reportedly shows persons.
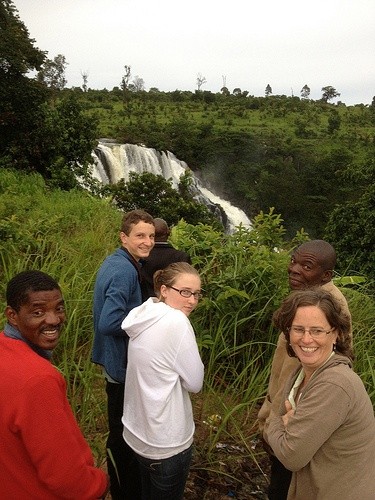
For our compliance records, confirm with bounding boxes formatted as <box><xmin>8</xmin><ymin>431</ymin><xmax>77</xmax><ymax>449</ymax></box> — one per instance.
<box><xmin>121</xmin><ymin>261</ymin><xmax>204</xmax><ymax>500</ymax></box>
<box><xmin>139</xmin><ymin>218</ymin><xmax>191</xmax><ymax>298</ymax></box>
<box><xmin>0</xmin><ymin>270</ymin><xmax>110</xmax><ymax>500</ymax></box>
<box><xmin>263</xmin><ymin>289</ymin><xmax>375</xmax><ymax>500</ymax></box>
<box><xmin>90</xmin><ymin>210</ymin><xmax>155</xmax><ymax>500</ymax></box>
<box><xmin>268</xmin><ymin>240</ymin><xmax>352</xmax><ymax>500</ymax></box>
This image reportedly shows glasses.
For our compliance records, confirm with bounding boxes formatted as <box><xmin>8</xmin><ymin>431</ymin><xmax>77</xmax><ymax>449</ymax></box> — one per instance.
<box><xmin>162</xmin><ymin>284</ymin><xmax>203</xmax><ymax>300</ymax></box>
<box><xmin>286</xmin><ymin>324</ymin><xmax>336</xmax><ymax>337</ymax></box>
